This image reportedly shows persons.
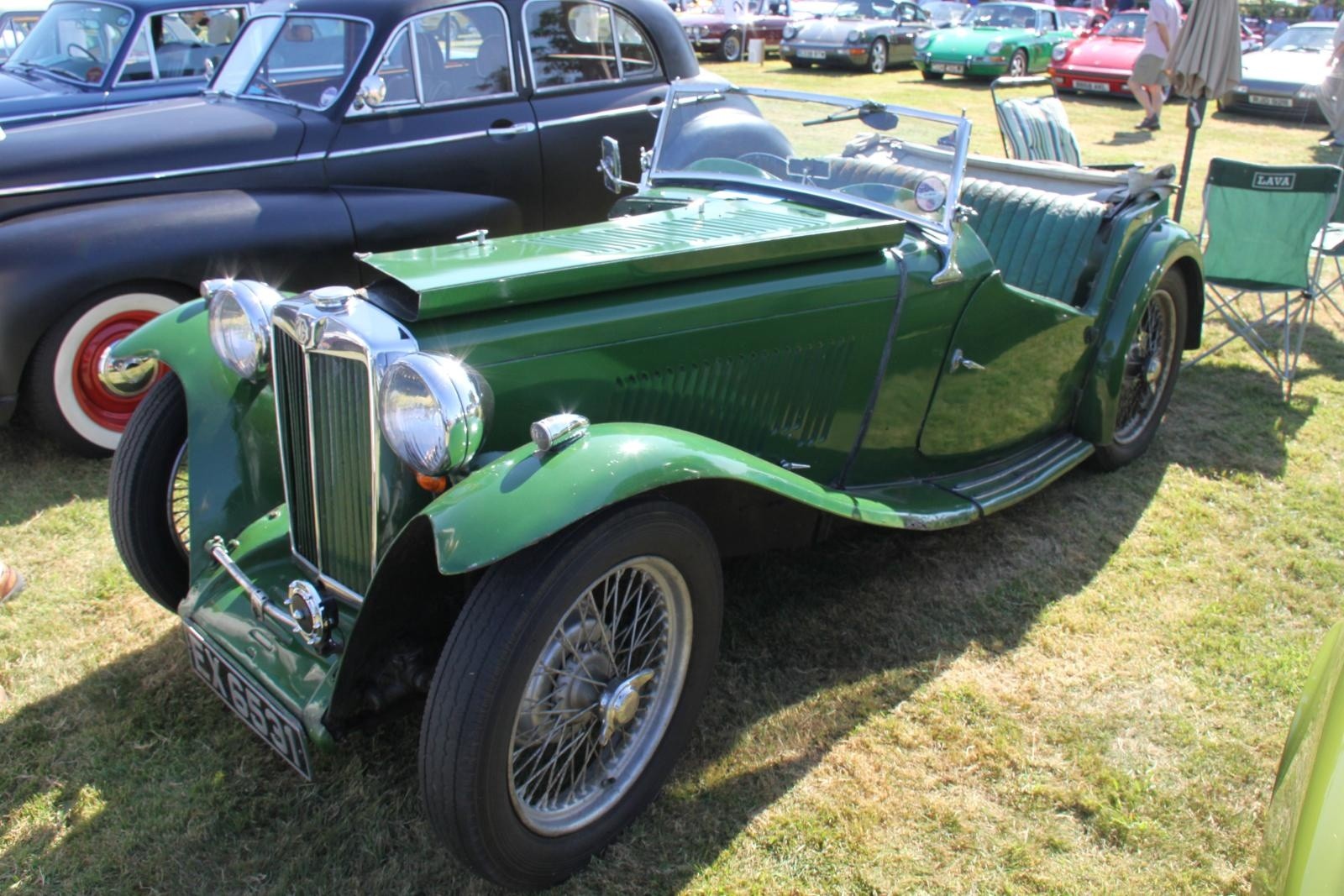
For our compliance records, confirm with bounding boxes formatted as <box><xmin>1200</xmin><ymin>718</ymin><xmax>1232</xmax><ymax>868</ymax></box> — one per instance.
<box><xmin>1264</xmin><ymin>7</ymin><xmax>1289</xmax><ymax>39</ymax></box>
<box><xmin>1315</xmin><ymin>11</ymin><xmax>1344</xmax><ymax>148</ymax></box>
<box><xmin>1127</xmin><ymin>0</ymin><xmax>1181</xmax><ymax>129</ymax></box>
<box><xmin>1307</xmin><ymin>0</ymin><xmax>1337</xmax><ymax>21</ymax></box>
<box><xmin>188</xmin><ymin>8</ymin><xmax>241</xmax><ymax>44</ymax></box>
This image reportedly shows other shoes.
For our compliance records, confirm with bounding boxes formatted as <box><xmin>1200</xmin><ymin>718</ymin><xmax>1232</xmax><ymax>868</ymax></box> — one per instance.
<box><xmin>1135</xmin><ymin>113</ymin><xmax>1159</xmax><ymax>129</ymax></box>
<box><xmin>1328</xmin><ymin>142</ymin><xmax>1344</xmax><ymax>147</ymax></box>
<box><xmin>1319</xmin><ymin>130</ymin><xmax>1334</xmax><ymax>141</ymax></box>
<box><xmin>1146</xmin><ymin>122</ymin><xmax>1160</xmax><ymax>131</ymax></box>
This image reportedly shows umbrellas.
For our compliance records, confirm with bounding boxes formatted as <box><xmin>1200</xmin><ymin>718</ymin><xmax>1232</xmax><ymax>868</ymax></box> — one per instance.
<box><xmin>1164</xmin><ymin>0</ymin><xmax>1242</xmax><ymax>223</ymax></box>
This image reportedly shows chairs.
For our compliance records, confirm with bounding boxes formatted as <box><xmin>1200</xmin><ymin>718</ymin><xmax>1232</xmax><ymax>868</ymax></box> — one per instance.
<box><xmin>460</xmin><ymin>37</ymin><xmax>507</xmax><ymax>99</ymax></box>
<box><xmin>385</xmin><ymin>34</ymin><xmax>451</xmax><ymax>102</ymax></box>
<box><xmin>1182</xmin><ymin>154</ymin><xmax>1344</xmax><ymax>412</ymax></box>
<box><xmin>155</xmin><ymin>42</ymin><xmax>191</xmax><ymax>78</ymax></box>
<box><xmin>989</xmin><ymin>74</ymin><xmax>1146</xmax><ymax>173</ymax></box>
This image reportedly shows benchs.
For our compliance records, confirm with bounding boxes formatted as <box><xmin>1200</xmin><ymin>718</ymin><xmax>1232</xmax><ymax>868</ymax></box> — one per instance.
<box><xmin>799</xmin><ymin>154</ymin><xmax>1105</xmax><ymax>302</ymax></box>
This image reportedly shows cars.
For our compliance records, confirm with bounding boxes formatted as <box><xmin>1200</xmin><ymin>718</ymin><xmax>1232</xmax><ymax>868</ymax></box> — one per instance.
<box><xmin>917</xmin><ymin>0</ymin><xmax>973</xmax><ymax>25</ymax></box>
<box><xmin>914</xmin><ymin>0</ymin><xmax>1076</xmax><ymax>87</ymax></box>
<box><xmin>779</xmin><ymin>0</ymin><xmax>938</xmax><ymax>75</ymax></box>
<box><xmin>677</xmin><ymin>0</ymin><xmax>816</xmax><ymax>64</ymax></box>
<box><xmin>1048</xmin><ymin>9</ymin><xmax>1188</xmax><ymax>105</ymax></box>
<box><xmin>1057</xmin><ymin>6</ymin><xmax>1134</xmax><ymax>38</ymax></box>
<box><xmin>109</xmin><ymin>79</ymin><xmax>1204</xmax><ymax>896</ymax></box>
<box><xmin>1240</xmin><ymin>21</ymin><xmax>1289</xmax><ymax>56</ymax></box>
<box><xmin>420</xmin><ymin>11</ymin><xmax>470</xmax><ymax>40</ymax></box>
<box><xmin>1</xmin><ymin>0</ymin><xmax>425</xmax><ymax>134</ymax></box>
<box><xmin>1</xmin><ymin>0</ymin><xmax>201</xmax><ymax>68</ymax></box>
<box><xmin>1216</xmin><ymin>20</ymin><xmax>1340</xmax><ymax>125</ymax></box>
<box><xmin>1</xmin><ymin>0</ymin><xmax>796</xmax><ymax>459</ymax></box>
